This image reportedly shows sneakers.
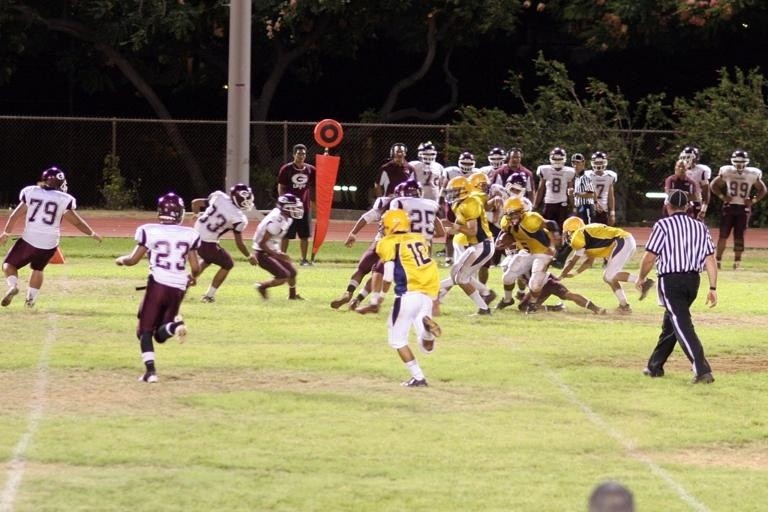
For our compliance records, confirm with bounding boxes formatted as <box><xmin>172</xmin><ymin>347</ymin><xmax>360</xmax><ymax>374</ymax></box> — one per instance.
<box><xmin>643</xmin><ymin>365</ymin><xmax>715</xmax><ymax>385</ymax></box>
<box><xmin>173</xmin><ymin>315</ymin><xmax>187</xmax><ymax>345</ymax></box>
<box><xmin>200</xmin><ymin>295</ymin><xmax>216</xmax><ymax>303</ymax></box>
<box><xmin>254</xmin><ymin>281</ymin><xmax>305</xmax><ymax>301</ymax></box>
<box><xmin>467</xmin><ymin>277</ymin><xmax>654</xmax><ymax>319</ymax></box>
<box><xmin>1</xmin><ymin>284</ymin><xmax>19</xmax><ymax>305</ymax></box>
<box><xmin>330</xmin><ymin>290</ymin><xmax>380</xmax><ymax>314</ymax></box>
<box><xmin>299</xmin><ymin>257</ymin><xmax>313</xmax><ymax>267</ymax></box>
<box><xmin>398</xmin><ymin>316</ymin><xmax>442</xmax><ymax>386</ymax></box>
<box><xmin>23</xmin><ymin>295</ymin><xmax>36</xmax><ymax>314</ymax></box>
<box><xmin>137</xmin><ymin>373</ymin><xmax>159</xmax><ymax>383</ymax></box>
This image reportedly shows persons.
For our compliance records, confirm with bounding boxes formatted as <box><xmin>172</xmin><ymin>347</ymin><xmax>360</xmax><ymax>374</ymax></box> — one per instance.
<box><xmin>373</xmin><ymin>142</ymin><xmax>534</xmax><ymax>301</ymax></box>
<box><xmin>185</xmin><ymin>181</ymin><xmax>259</xmax><ymax>305</ymax></box>
<box><xmin>534</xmin><ymin>148</ymin><xmax>618</xmax><ymax>265</ymax></box>
<box><xmin>251</xmin><ymin>193</ymin><xmax>308</xmax><ymax>302</ymax></box>
<box><xmin>275</xmin><ymin>144</ymin><xmax>316</xmax><ymax>267</ymax></box>
<box><xmin>330</xmin><ymin>173</ymin><xmax>654</xmax><ymax>315</ymax></box>
<box><xmin>635</xmin><ymin>190</ymin><xmax>718</xmax><ymax>385</ymax></box>
<box><xmin>0</xmin><ymin>163</ymin><xmax>105</xmax><ymax>309</ymax></box>
<box><xmin>373</xmin><ymin>207</ymin><xmax>442</xmax><ymax>388</ymax></box>
<box><xmin>587</xmin><ymin>481</ymin><xmax>633</xmax><ymax>511</ymax></box>
<box><xmin>115</xmin><ymin>190</ymin><xmax>201</xmax><ymax>383</ymax></box>
<box><xmin>663</xmin><ymin>147</ymin><xmax>712</xmax><ymax>222</ymax></box>
<box><xmin>710</xmin><ymin>151</ymin><xmax>768</xmax><ymax>268</ymax></box>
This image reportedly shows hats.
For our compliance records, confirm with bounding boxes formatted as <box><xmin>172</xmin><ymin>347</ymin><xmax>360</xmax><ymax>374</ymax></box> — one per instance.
<box><xmin>666</xmin><ymin>188</ymin><xmax>690</xmax><ymax>209</ymax></box>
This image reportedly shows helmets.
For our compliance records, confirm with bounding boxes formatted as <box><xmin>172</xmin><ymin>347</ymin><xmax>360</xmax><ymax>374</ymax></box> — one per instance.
<box><xmin>276</xmin><ymin>194</ymin><xmax>305</xmax><ymax>220</ymax></box>
<box><xmin>416</xmin><ymin>140</ymin><xmax>439</xmax><ymax>169</ymax></box>
<box><xmin>678</xmin><ymin>145</ymin><xmax>751</xmax><ymax>176</ymax></box>
<box><xmin>379</xmin><ymin>180</ymin><xmax>423</xmax><ymax>237</ymax></box>
<box><xmin>228</xmin><ymin>183</ymin><xmax>255</xmax><ymax>211</ymax></box>
<box><xmin>156</xmin><ymin>194</ymin><xmax>185</xmax><ymax>225</ymax></box>
<box><xmin>443</xmin><ymin>148</ymin><xmax>608</xmax><ymax>246</ymax></box>
<box><xmin>41</xmin><ymin>167</ymin><xmax>65</xmax><ymax>190</ymax></box>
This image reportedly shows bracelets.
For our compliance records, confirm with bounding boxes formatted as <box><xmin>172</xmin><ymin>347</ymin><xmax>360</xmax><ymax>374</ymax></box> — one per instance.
<box><xmin>2</xmin><ymin>231</ymin><xmax>10</xmax><ymax>238</ymax></box>
<box><xmin>379</xmin><ymin>289</ymin><xmax>388</xmax><ymax>299</ymax></box>
<box><xmin>192</xmin><ymin>212</ymin><xmax>199</xmax><ymax>219</ymax></box>
<box><xmin>709</xmin><ymin>286</ymin><xmax>716</xmax><ymax>291</ymax></box>
<box><xmin>190</xmin><ymin>273</ymin><xmax>197</xmax><ymax>280</ymax></box>
<box><xmin>90</xmin><ymin>231</ymin><xmax>96</xmax><ymax>237</ymax></box>
<box><xmin>276</xmin><ymin>250</ymin><xmax>283</xmax><ymax>260</ymax></box>
<box><xmin>637</xmin><ymin>274</ymin><xmax>647</xmax><ymax>285</ymax></box>
<box><xmin>246</xmin><ymin>253</ymin><xmax>253</xmax><ymax>261</ymax></box>
